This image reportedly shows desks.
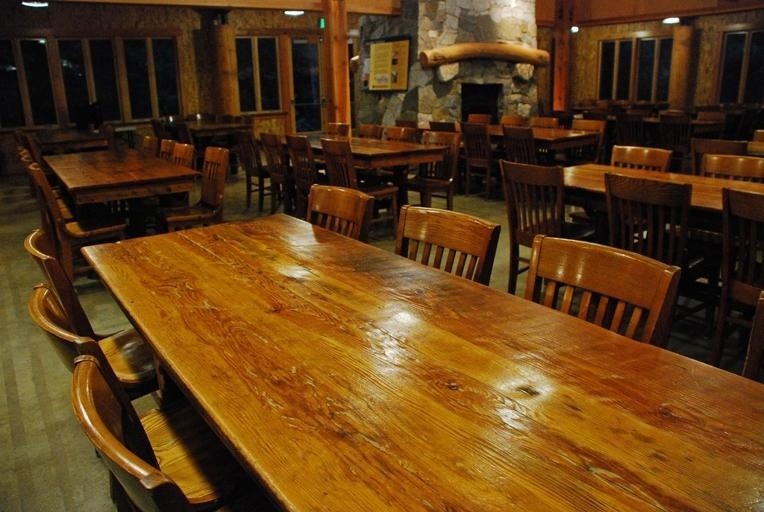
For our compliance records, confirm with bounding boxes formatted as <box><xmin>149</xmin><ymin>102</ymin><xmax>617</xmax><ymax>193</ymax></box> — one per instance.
<box><xmin>563</xmin><ymin>163</ymin><xmax>764</xmax><ymax>215</ymax></box>
<box><xmin>41</xmin><ymin>148</ymin><xmax>201</xmax><ymax>205</ymax></box>
<box><xmin>486</xmin><ymin>124</ymin><xmax>600</xmax><ymax>151</ymax></box>
<box><xmin>80</xmin><ymin>212</ymin><xmax>764</xmax><ymax>511</ymax></box>
<box><xmin>274</xmin><ymin>131</ymin><xmax>448</xmax><ymax>170</ymax></box>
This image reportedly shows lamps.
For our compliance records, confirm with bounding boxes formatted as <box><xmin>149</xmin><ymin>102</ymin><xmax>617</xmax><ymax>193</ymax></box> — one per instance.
<box><xmin>22</xmin><ymin>1</ymin><xmax>48</xmax><ymax>7</ymax></box>
<box><xmin>283</xmin><ymin>11</ymin><xmax>304</xmax><ymax>16</ymax></box>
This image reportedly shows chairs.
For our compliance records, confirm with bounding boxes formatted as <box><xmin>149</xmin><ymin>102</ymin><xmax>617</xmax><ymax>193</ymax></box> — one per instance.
<box><xmin>171</xmin><ymin>143</ymin><xmax>193</xmax><ymax>208</ymax></box>
<box><xmin>713</xmin><ymin>188</ymin><xmax>763</xmax><ymax>363</ymax></box>
<box><xmin>386</xmin><ymin>127</ymin><xmax>415</xmax><ymax>141</ymax></box>
<box><xmin>71</xmin><ymin>355</ymin><xmax>246</xmax><ymax>512</ymax></box>
<box><xmin>523</xmin><ymin>234</ymin><xmax>681</xmax><ymax>343</ymax></box>
<box><xmin>326</xmin><ymin>123</ymin><xmax>348</xmax><ymax>136</ymax></box>
<box><xmin>610</xmin><ymin>145</ymin><xmax>673</xmax><ymax>172</ymax></box>
<box><xmin>306</xmin><ymin>184</ymin><xmax>375</xmax><ymax>243</ymax></box>
<box><xmin>402</xmin><ymin>131</ymin><xmax>461</xmax><ymax>210</ymax></box>
<box><xmin>571</xmin><ymin>120</ymin><xmax>606</xmax><ymax>163</ymax></box>
<box><xmin>459</xmin><ymin>122</ymin><xmax>505</xmax><ymax>201</ymax></box>
<box><xmin>259</xmin><ymin>133</ymin><xmax>326</xmax><ymax>218</ymax></box>
<box><xmin>499</xmin><ymin>159</ymin><xmax>598</xmax><ymax>295</ymax></box>
<box><xmin>162</xmin><ymin>146</ymin><xmax>228</xmax><ymax>233</ymax></box>
<box><xmin>395</xmin><ymin>121</ymin><xmax>417</xmax><ymax>134</ymax></box>
<box><xmin>320</xmin><ymin>138</ymin><xmax>398</xmax><ymax>238</ymax></box>
<box><xmin>500</xmin><ymin>124</ymin><xmax>563</xmax><ymax>204</ymax></box>
<box><xmin>466</xmin><ymin>114</ymin><xmax>491</xmax><ymax>125</ymax></box>
<box><xmin>529</xmin><ymin>117</ymin><xmax>559</xmax><ymax>128</ymax></box>
<box><xmin>25</xmin><ymin>227</ymin><xmax>125</xmax><ymax>340</ymax></box>
<box><xmin>501</xmin><ymin>116</ymin><xmax>521</xmax><ymax>126</ymax></box>
<box><xmin>690</xmin><ymin>138</ymin><xmax>748</xmax><ymax>176</ymax></box>
<box><xmin>359</xmin><ymin>125</ymin><xmax>383</xmax><ymax>140</ymax></box>
<box><xmin>19</xmin><ymin>149</ymin><xmax>127</xmax><ymax>286</ymax></box>
<box><xmin>142</xmin><ymin>134</ymin><xmax>175</xmax><ymax>158</ymax></box>
<box><xmin>394</xmin><ymin>205</ymin><xmax>501</xmax><ymax>286</ymax></box>
<box><xmin>28</xmin><ymin>284</ymin><xmax>162</xmax><ymax>401</ymax></box>
<box><xmin>603</xmin><ymin>172</ymin><xmax>721</xmax><ymax>347</ymax></box>
<box><xmin>237</xmin><ymin>130</ymin><xmax>290</xmax><ymax>211</ymax></box>
<box><xmin>700</xmin><ymin>154</ymin><xmax>764</xmax><ymax>183</ymax></box>
<box><xmin>428</xmin><ymin>121</ymin><xmax>455</xmax><ymax>132</ymax></box>
